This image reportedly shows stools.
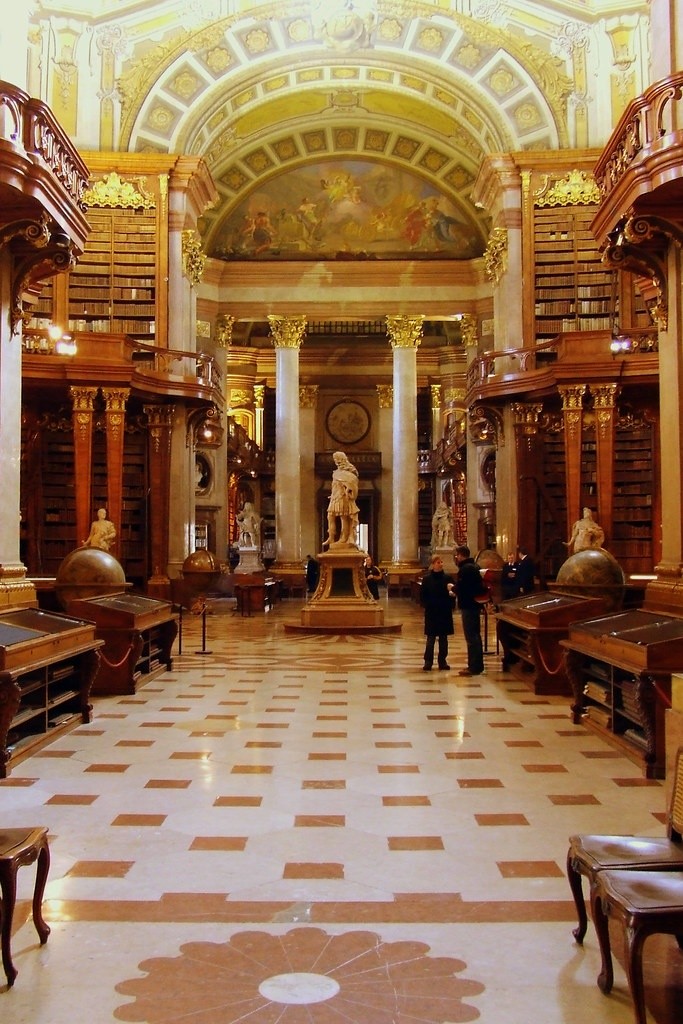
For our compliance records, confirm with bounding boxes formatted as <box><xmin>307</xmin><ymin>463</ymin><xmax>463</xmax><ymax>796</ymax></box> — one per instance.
<box><xmin>0</xmin><ymin>824</ymin><xmax>50</xmax><ymax>986</ymax></box>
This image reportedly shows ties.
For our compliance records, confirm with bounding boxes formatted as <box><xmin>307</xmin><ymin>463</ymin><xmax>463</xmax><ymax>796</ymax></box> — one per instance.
<box><xmin>510</xmin><ymin>563</ymin><xmax>512</xmax><ymax>570</ymax></box>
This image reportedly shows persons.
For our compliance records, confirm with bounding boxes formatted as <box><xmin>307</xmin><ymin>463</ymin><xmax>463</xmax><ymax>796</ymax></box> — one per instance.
<box><xmin>434</xmin><ymin>502</ymin><xmax>452</xmax><ymax>547</ymax></box>
<box><xmin>227</xmin><ymin>548</ymin><xmax>240</xmax><ymax>569</ymax></box>
<box><xmin>82</xmin><ymin>509</ymin><xmax>116</xmax><ymax>551</ymax></box>
<box><xmin>562</xmin><ymin>507</ymin><xmax>603</xmax><ymax>553</ymax></box>
<box><xmin>195</xmin><ymin>464</ymin><xmax>202</xmax><ymax>487</ymax></box>
<box><xmin>501</xmin><ymin>548</ymin><xmax>534</xmax><ymax>600</ymax></box>
<box><xmin>419</xmin><ymin>554</ymin><xmax>454</xmax><ymax>670</ymax></box>
<box><xmin>305</xmin><ymin>555</ymin><xmax>319</xmax><ymax>592</ymax></box>
<box><xmin>236</xmin><ymin>502</ymin><xmax>263</xmax><ymax>547</ymax></box>
<box><xmin>322</xmin><ymin>452</ymin><xmax>359</xmax><ymax>545</ymax></box>
<box><xmin>364</xmin><ymin>555</ymin><xmax>382</xmax><ymax>600</ymax></box>
<box><xmin>447</xmin><ymin>544</ymin><xmax>486</xmax><ymax>675</ymax></box>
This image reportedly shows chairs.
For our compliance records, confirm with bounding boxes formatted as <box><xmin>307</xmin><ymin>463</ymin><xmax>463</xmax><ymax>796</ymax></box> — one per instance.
<box><xmin>565</xmin><ymin>746</ymin><xmax>683</xmax><ymax>1024</ymax></box>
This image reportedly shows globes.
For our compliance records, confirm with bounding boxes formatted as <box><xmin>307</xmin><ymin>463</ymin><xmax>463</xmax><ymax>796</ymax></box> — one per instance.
<box><xmin>474</xmin><ymin>549</ymin><xmax>504</xmax><ymax>569</ymax></box>
<box><xmin>49</xmin><ymin>546</ymin><xmax>133</xmax><ymax>611</ymax></box>
<box><xmin>178</xmin><ymin>547</ymin><xmax>222</xmax><ymax>613</ymax></box>
<box><xmin>555</xmin><ymin>546</ymin><xmax>625</xmax><ymax>585</ymax></box>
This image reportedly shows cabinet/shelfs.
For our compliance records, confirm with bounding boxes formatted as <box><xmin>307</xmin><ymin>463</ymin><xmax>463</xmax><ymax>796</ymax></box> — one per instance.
<box><xmin>0</xmin><ymin>638</ymin><xmax>104</xmax><ymax>778</ymax></box>
<box><xmin>90</xmin><ymin>613</ymin><xmax>183</xmax><ymax>696</ymax></box>
<box><xmin>494</xmin><ymin>612</ymin><xmax>575</xmax><ymax>697</ymax></box>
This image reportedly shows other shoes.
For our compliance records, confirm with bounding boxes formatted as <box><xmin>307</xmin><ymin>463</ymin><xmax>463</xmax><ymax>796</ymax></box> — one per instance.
<box><xmin>439</xmin><ymin>665</ymin><xmax>450</xmax><ymax>670</ymax></box>
<box><xmin>458</xmin><ymin>668</ymin><xmax>480</xmax><ymax>675</ymax></box>
<box><xmin>423</xmin><ymin>665</ymin><xmax>431</xmax><ymax>670</ymax></box>
